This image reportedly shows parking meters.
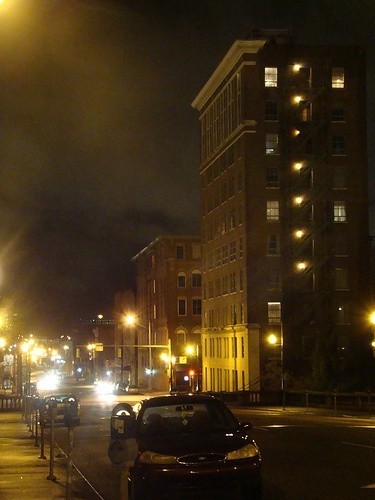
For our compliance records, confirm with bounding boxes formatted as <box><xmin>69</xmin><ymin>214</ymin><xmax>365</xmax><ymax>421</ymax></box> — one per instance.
<box><xmin>63</xmin><ymin>397</ymin><xmax>80</xmax><ymax>498</ymax></box>
<box><xmin>26</xmin><ymin>396</ymin><xmax>47</xmax><ymax>459</ymax></box>
<box><xmin>47</xmin><ymin>397</ymin><xmax>60</xmax><ymax>480</ymax></box>
<box><xmin>108</xmin><ymin>402</ymin><xmax>138</xmax><ymax>500</ymax></box>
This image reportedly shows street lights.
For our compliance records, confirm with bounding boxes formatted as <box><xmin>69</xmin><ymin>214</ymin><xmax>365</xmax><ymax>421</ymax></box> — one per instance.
<box><xmin>124</xmin><ymin>313</ymin><xmax>153</xmax><ymax>389</ymax></box>
<box><xmin>268</xmin><ymin>334</ymin><xmax>283</xmax><ymax>406</ymax></box>
<box><xmin>186</xmin><ymin>344</ymin><xmax>199</xmax><ymax>392</ymax></box>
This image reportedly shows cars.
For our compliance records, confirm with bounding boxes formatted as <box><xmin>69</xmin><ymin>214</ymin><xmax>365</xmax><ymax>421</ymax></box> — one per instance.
<box><xmin>127</xmin><ymin>393</ymin><xmax>263</xmax><ymax>500</ymax></box>
<box><xmin>40</xmin><ymin>393</ymin><xmax>81</xmax><ymax>427</ymax></box>
<box><xmin>34</xmin><ymin>367</ymin><xmax>133</xmax><ymax>395</ymax></box>
